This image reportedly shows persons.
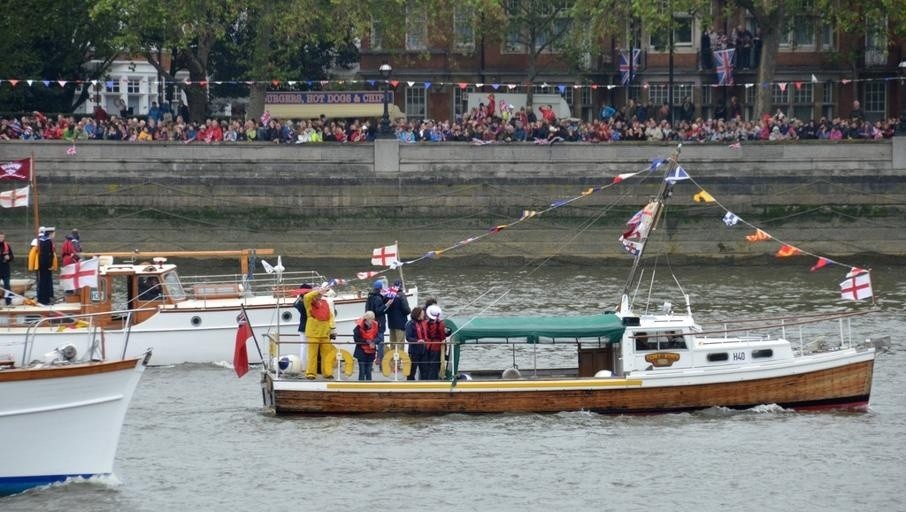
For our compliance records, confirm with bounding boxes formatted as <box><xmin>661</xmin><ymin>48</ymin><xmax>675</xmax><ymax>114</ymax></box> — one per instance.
<box><xmin>0</xmin><ymin>231</ymin><xmax>15</xmax><ymax>305</ymax></box>
<box><xmin>28</xmin><ymin>224</ymin><xmax>82</xmax><ymax>304</ymax></box>
<box><xmin>293</xmin><ymin>281</ymin><xmax>446</xmax><ymax>381</ymax></box>
<box><xmin>0</xmin><ymin>92</ymin><xmax>904</xmax><ymax>143</ymax></box>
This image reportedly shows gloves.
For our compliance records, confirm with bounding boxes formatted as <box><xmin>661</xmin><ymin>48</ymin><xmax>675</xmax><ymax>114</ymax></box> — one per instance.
<box><xmin>330</xmin><ymin>332</ymin><xmax>338</xmax><ymax>340</ymax></box>
<box><xmin>319</xmin><ymin>286</ymin><xmax>330</xmax><ymax>295</ymax></box>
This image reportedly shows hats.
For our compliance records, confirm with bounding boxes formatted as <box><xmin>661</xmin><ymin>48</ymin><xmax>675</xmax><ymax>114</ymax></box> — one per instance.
<box><xmin>321</xmin><ymin>282</ymin><xmax>336</xmax><ymax>296</ymax></box>
<box><xmin>38</xmin><ymin>226</ymin><xmax>55</xmax><ymax>238</ymax></box>
<box><xmin>364</xmin><ymin>311</ymin><xmax>375</xmax><ymax>320</ymax></box>
<box><xmin>300</xmin><ymin>283</ymin><xmax>312</xmax><ymax>289</ymax></box>
<box><xmin>374</xmin><ymin>280</ymin><xmax>384</xmax><ymax>288</ymax></box>
<box><xmin>426</xmin><ymin>305</ymin><xmax>442</xmax><ymax>320</ymax></box>
<box><xmin>394</xmin><ymin>280</ymin><xmax>400</xmax><ymax>286</ymax></box>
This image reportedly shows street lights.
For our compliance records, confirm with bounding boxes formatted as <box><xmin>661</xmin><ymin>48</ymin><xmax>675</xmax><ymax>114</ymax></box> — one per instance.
<box><xmin>379</xmin><ymin>64</ymin><xmax>393</xmax><ymax>139</ymax></box>
<box><xmin>87</xmin><ymin>72</ymin><xmax>112</xmax><ymax>107</ymax></box>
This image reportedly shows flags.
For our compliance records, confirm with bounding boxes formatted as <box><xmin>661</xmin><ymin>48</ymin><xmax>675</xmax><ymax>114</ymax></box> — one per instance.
<box><xmin>371</xmin><ymin>244</ymin><xmax>397</xmax><ymax>267</ymax></box>
<box><xmin>839</xmin><ymin>271</ymin><xmax>872</xmax><ymax>301</ymax></box>
<box><xmin>233</xmin><ymin>312</ymin><xmax>253</xmax><ymax>378</ymax></box>
<box><xmin>619</xmin><ymin>49</ymin><xmax>641</xmax><ymax>87</ymax></box>
<box><xmin>0</xmin><ymin>185</ymin><xmax>29</xmax><ymax>209</ymax></box>
<box><xmin>59</xmin><ymin>255</ymin><xmax>97</xmax><ymax>292</ymax></box>
<box><xmin>714</xmin><ymin>49</ymin><xmax>734</xmax><ymax>85</ymax></box>
<box><xmin>618</xmin><ymin>201</ymin><xmax>657</xmax><ymax>255</ymax></box>
<box><xmin>0</xmin><ymin>155</ymin><xmax>31</xmax><ymax>182</ymax></box>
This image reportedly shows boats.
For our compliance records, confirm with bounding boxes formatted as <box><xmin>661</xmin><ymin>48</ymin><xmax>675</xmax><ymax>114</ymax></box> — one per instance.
<box><xmin>0</xmin><ymin>150</ymin><xmax>420</xmax><ymax>374</ymax></box>
<box><xmin>0</xmin><ymin>307</ymin><xmax>159</xmax><ymax>497</ymax></box>
<box><xmin>232</xmin><ymin>143</ymin><xmax>884</xmax><ymax>417</ymax></box>
